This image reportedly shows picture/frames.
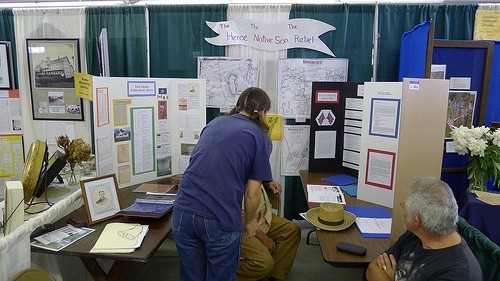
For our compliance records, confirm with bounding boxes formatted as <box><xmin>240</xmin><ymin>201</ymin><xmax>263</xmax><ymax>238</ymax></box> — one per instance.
<box><xmin>26</xmin><ymin>38</ymin><xmax>84</xmax><ymax>121</ymax></box>
<box><xmin>80</xmin><ymin>174</ymin><xmax>123</xmax><ymax>227</ymax></box>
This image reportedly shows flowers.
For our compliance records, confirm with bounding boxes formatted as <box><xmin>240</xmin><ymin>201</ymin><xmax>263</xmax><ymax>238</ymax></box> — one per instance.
<box><xmin>55</xmin><ymin>134</ymin><xmax>91</xmax><ymax>183</ymax></box>
<box><xmin>449</xmin><ymin>124</ymin><xmax>500</xmax><ymax>189</ymax></box>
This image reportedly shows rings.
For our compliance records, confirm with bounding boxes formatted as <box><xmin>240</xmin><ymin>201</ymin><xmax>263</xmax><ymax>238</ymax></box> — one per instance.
<box><xmin>382</xmin><ymin>266</ymin><xmax>386</xmax><ymax>270</ymax></box>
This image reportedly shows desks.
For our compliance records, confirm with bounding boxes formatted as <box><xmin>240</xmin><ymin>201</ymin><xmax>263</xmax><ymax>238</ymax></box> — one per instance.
<box><xmin>299</xmin><ymin>170</ymin><xmax>394</xmax><ymax>269</ymax></box>
<box><xmin>30</xmin><ymin>173</ymin><xmax>183</xmax><ymax>281</ymax></box>
<box><xmin>456</xmin><ymin>190</ymin><xmax>500</xmax><ymax>248</ymax></box>
<box><xmin>0</xmin><ymin>172</ymin><xmax>115</xmax><ymax>281</ymax></box>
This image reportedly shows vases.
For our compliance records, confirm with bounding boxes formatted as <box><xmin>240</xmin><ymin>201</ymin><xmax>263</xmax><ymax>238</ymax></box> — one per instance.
<box><xmin>468</xmin><ymin>170</ymin><xmax>488</xmax><ymax>194</ymax></box>
<box><xmin>67</xmin><ymin>165</ymin><xmax>80</xmax><ymax>186</ymax></box>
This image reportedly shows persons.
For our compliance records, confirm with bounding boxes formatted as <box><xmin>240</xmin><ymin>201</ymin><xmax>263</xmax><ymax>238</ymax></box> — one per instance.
<box><xmin>171</xmin><ymin>87</ymin><xmax>274</xmax><ymax>281</ymax></box>
<box><xmin>362</xmin><ymin>176</ymin><xmax>484</xmax><ymax>281</ymax></box>
<box><xmin>239</xmin><ymin>122</ymin><xmax>302</xmax><ymax>281</ymax></box>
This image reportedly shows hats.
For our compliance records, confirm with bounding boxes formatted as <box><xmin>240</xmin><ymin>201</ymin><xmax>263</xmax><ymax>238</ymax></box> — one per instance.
<box><xmin>306</xmin><ymin>203</ymin><xmax>357</xmax><ymax>231</ymax></box>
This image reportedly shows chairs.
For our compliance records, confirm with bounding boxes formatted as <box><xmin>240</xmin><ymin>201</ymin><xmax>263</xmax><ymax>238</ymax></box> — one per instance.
<box><xmin>455</xmin><ymin>216</ymin><xmax>500</xmax><ymax>281</ymax></box>
<box><xmin>261</xmin><ymin>185</ymin><xmax>281</xmax><ymax>218</ymax></box>
<box><xmin>8</xmin><ymin>267</ymin><xmax>57</xmax><ymax>281</ymax></box>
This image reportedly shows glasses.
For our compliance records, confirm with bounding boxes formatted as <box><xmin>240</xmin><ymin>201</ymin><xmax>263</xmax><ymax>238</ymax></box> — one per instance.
<box><xmin>117</xmin><ymin>224</ymin><xmax>143</xmax><ymax>240</ymax></box>
